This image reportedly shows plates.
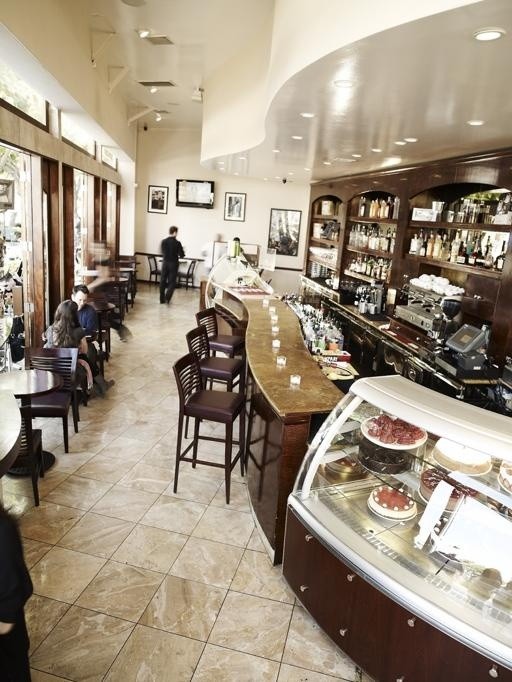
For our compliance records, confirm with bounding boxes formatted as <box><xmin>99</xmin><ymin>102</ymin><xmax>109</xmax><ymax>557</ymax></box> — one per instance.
<box><xmin>429</xmin><ymin>538</ymin><xmax>468</xmax><ymax>566</ymax></box>
<box><xmin>361</xmin><ymin>415</ymin><xmax>428</xmax><ymax>450</ymax></box>
<box><xmin>498</xmin><ymin>472</ymin><xmax>512</xmax><ymax>495</ymax></box>
<box><xmin>367</xmin><ymin>501</ymin><xmax>418</xmax><ymax>523</ymax></box>
<box><xmin>417</xmin><ymin>486</ymin><xmax>455</xmax><ymax>513</ymax></box>
<box><xmin>432</xmin><ymin>446</ymin><xmax>492</xmax><ymax>476</ymax></box>
<box><xmin>357</xmin><ymin>456</ymin><xmax>414</xmax><ymax>477</ymax></box>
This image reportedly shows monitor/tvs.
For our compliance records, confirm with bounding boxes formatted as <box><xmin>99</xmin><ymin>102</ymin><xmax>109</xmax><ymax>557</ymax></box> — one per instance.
<box><xmin>446</xmin><ymin>323</ymin><xmax>486</xmax><ymax>354</ymax></box>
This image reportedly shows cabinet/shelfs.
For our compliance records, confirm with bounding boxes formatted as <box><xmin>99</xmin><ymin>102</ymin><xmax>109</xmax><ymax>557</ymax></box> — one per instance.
<box><xmin>298</xmin><ymin>146</ymin><xmax>512</xmax><ymax>399</ymax></box>
<box><xmin>283</xmin><ymin>374</ymin><xmax>512</xmax><ymax>682</ymax></box>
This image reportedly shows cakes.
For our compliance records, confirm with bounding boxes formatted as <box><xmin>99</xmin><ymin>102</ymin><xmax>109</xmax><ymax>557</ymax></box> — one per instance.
<box><xmin>433</xmin><ymin>437</ymin><xmax>492</xmax><ymax>474</ymax></box>
<box><xmin>499</xmin><ymin>460</ymin><xmax>512</xmax><ymax>494</ymax></box>
<box><xmin>420</xmin><ymin>468</ymin><xmax>479</xmax><ymax>511</ymax></box>
<box><xmin>368</xmin><ymin>484</ymin><xmax>417</xmax><ymax>520</ymax></box>
<box><xmin>357</xmin><ymin>436</ymin><xmax>412</xmax><ymax>474</ymax></box>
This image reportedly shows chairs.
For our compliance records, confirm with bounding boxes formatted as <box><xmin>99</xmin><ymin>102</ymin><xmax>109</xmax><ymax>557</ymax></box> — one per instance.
<box><xmin>178</xmin><ymin>260</ymin><xmax>196</xmax><ymax>290</ymax></box>
<box><xmin>173</xmin><ymin>351</ymin><xmax>247</xmax><ymax>504</ymax></box>
<box><xmin>185</xmin><ymin>323</ymin><xmax>245</xmax><ymax>440</ymax></box>
<box><xmin>122</xmin><ymin>273</ymin><xmax>131</xmax><ymax>312</ymax></box>
<box><xmin>23</xmin><ymin>346</ymin><xmax>79</xmax><ymax>452</ymax></box>
<box><xmin>148</xmin><ymin>255</ymin><xmax>164</xmax><ymax>287</ymax></box>
<box><xmin>195</xmin><ymin>306</ymin><xmax>245</xmax><ymax>390</ymax></box>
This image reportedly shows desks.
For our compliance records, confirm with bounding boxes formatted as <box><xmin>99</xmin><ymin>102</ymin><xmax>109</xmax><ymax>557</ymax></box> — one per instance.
<box><xmin>0</xmin><ymin>369</ymin><xmax>63</xmax><ymax>477</ymax></box>
<box><xmin>86</xmin><ymin>300</ymin><xmax>115</xmax><ymax>352</ymax></box>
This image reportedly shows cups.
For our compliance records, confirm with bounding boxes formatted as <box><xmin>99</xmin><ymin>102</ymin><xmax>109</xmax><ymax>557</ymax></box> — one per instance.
<box><xmin>268</xmin><ymin>306</ymin><xmax>276</xmax><ymax>314</ymax></box>
<box><xmin>276</xmin><ymin>356</ymin><xmax>287</xmax><ymax>367</ymax></box>
<box><xmin>263</xmin><ymin>299</ymin><xmax>269</xmax><ymax>307</ymax></box>
<box><xmin>272</xmin><ymin>340</ymin><xmax>280</xmax><ymax>348</ymax></box>
<box><xmin>291</xmin><ymin>374</ymin><xmax>301</xmax><ymax>385</ymax></box>
<box><xmin>272</xmin><ymin>324</ymin><xmax>279</xmax><ymax>334</ymax></box>
<box><xmin>271</xmin><ymin>313</ymin><xmax>279</xmax><ymax>323</ymax></box>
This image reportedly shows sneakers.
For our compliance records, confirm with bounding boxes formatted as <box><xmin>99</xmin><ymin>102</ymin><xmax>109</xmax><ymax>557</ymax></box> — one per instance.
<box><xmin>98</xmin><ymin>379</ymin><xmax>115</xmax><ymax>394</ymax></box>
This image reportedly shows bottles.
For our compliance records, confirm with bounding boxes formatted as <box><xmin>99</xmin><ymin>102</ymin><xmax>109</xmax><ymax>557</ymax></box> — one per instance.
<box><xmin>347</xmin><ymin>254</ymin><xmax>392</xmax><ymax>282</ymax></box>
<box><xmin>408</xmin><ymin>228</ymin><xmax>494</xmax><ymax>269</ymax></box>
<box><xmin>494</xmin><ymin>240</ymin><xmax>506</xmax><ymax>271</ymax></box>
<box><xmin>232</xmin><ymin>237</ymin><xmax>241</xmax><ymax>258</ymax></box>
<box><xmin>361</xmin><ymin>289</ymin><xmax>366</xmax><ymax>302</ymax></box>
<box><xmin>347</xmin><ymin>224</ymin><xmax>396</xmax><ymax>254</ymax></box>
<box><xmin>357</xmin><ymin>195</ymin><xmax>400</xmax><ymax>220</ymax></box>
<box><xmin>353</xmin><ymin>290</ymin><xmax>361</xmax><ymax>306</ymax></box>
<box><xmin>335</xmin><ymin>328</ymin><xmax>345</xmax><ymax>350</ymax></box>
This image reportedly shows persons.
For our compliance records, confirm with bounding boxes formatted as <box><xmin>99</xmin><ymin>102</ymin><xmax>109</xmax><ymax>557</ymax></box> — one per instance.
<box><xmin>159</xmin><ymin>226</ymin><xmax>186</xmax><ymax>304</ymax></box>
<box><xmin>71</xmin><ymin>285</ymin><xmax>96</xmax><ymax>332</ymax></box>
<box><xmin>41</xmin><ymin>300</ymin><xmax>115</xmax><ymax>393</ymax></box>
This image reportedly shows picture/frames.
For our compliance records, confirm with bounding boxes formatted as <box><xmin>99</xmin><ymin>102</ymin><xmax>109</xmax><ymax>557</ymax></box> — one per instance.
<box><xmin>148</xmin><ymin>184</ymin><xmax>170</xmax><ymax>214</ymax></box>
<box><xmin>224</xmin><ymin>191</ymin><xmax>246</xmax><ymax>222</ymax></box>
<box><xmin>267</xmin><ymin>207</ymin><xmax>301</xmax><ymax>257</ymax></box>
<box><xmin>213</xmin><ymin>242</ymin><xmax>258</xmax><ymax>269</ymax></box>
